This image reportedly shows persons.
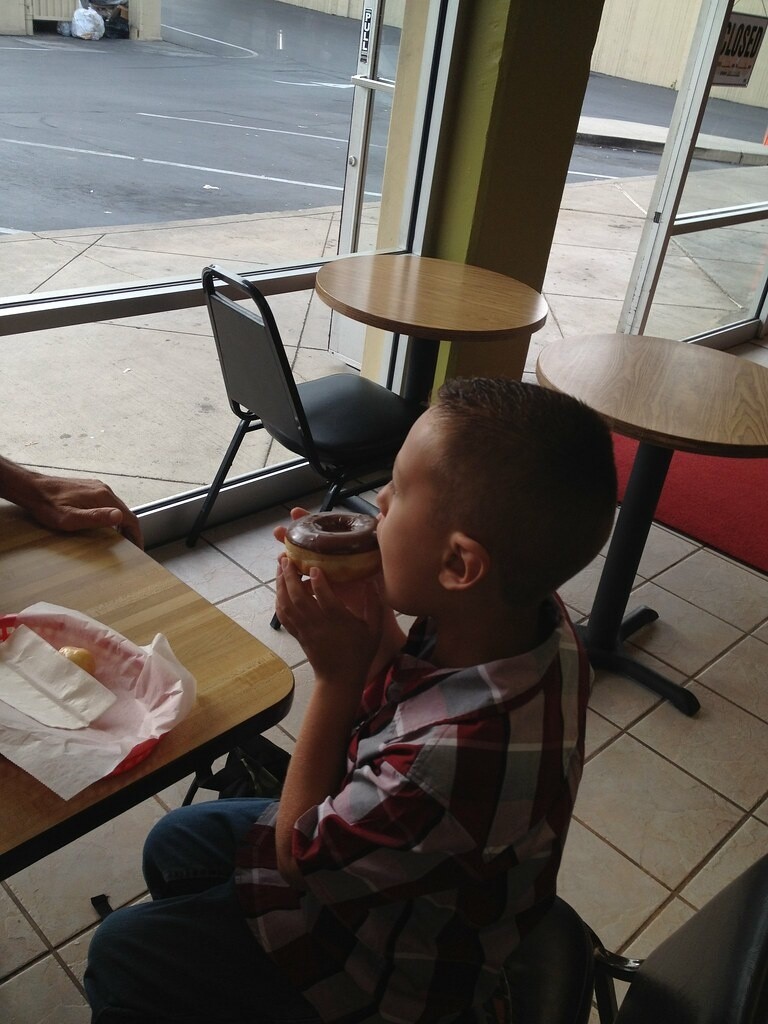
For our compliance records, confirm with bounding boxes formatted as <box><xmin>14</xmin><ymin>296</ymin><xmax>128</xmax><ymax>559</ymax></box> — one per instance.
<box><xmin>82</xmin><ymin>377</ymin><xmax>618</xmax><ymax>1023</ymax></box>
<box><xmin>0</xmin><ymin>455</ymin><xmax>143</xmax><ymax>550</ymax></box>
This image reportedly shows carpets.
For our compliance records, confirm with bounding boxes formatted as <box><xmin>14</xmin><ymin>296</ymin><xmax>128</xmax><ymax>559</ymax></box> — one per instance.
<box><xmin>608</xmin><ymin>429</ymin><xmax>766</xmax><ymax>579</ymax></box>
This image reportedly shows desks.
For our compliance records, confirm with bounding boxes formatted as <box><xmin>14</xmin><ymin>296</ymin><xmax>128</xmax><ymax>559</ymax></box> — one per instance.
<box><xmin>536</xmin><ymin>333</ymin><xmax>767</xmax><ymax>716</ymax></box>
<box><xmin>315</xmin><ymin>252</ymin><xmax>548</xmax><ymax>525</ymax></box>
<box><xmin>1</xmin><ymin>496</ymin><xmax>294</xmax><ymax>891</ymax></box>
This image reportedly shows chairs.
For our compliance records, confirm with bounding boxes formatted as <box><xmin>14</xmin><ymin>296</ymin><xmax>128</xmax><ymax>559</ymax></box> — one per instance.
<box><xmin>509</xmin><ymin>852</ymin><xmax>768</xmax><ymax>1024</ymax></box>
<box><xmin>186</xmin><ymin>266</ymin><xmax>429</xmax><ymax>627</ymax></box>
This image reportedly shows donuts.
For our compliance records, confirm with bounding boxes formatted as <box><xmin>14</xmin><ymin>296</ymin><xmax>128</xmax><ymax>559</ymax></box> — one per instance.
<box><xmin>283</xmin><ymin>511</ymin><xmax>382</xmax><ymax>583</ymax></box>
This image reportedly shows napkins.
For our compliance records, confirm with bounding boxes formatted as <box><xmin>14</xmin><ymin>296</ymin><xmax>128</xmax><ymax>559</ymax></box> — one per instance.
<box><xmin>0</xmin><ymin>624</ymin><xmax>118</xmax><ymax>729</ymax></box>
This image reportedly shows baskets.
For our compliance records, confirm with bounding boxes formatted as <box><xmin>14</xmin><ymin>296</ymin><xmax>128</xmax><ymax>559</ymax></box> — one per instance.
<box><xmin>0</xmin><ymin>608</ymin><xmax>172</xmax><ymax>780</ymax></box>
